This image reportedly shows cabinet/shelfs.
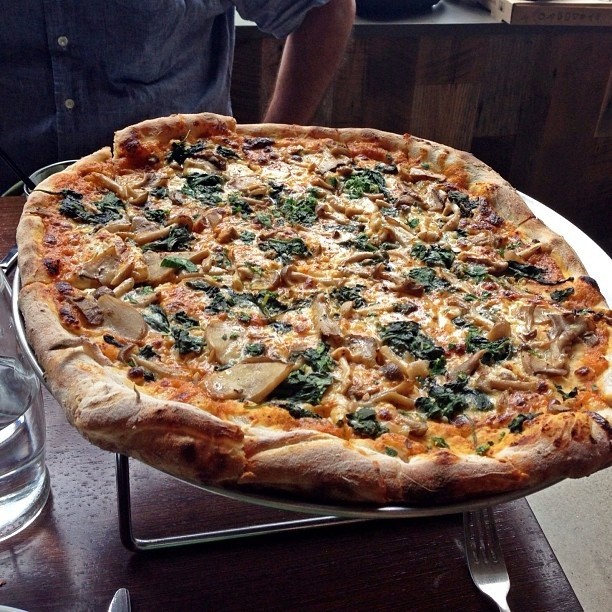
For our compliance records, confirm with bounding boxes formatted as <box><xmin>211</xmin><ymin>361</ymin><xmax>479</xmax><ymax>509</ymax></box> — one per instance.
<box><xmin>479</xmin><ymin>28</ymin><xmax>611</xmax><ymax>250</ymax></box>
<box><xmin>261</xmin><ymin>22</ymin><xmax>477</xmax><ymax>153</ymax></box>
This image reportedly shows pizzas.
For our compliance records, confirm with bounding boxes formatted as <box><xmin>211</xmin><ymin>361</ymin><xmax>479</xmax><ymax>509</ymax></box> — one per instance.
<box><xmin>16</xmin><ymin>109</ymin><xmax>611</xmax><ymax>504</ymax></box>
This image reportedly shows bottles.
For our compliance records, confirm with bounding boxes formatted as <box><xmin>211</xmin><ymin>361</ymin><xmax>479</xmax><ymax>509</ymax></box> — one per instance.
<box><xmin>1</xmin><ymin>261</ymin><xmax>51</xmax><ymax>545</ymax></box>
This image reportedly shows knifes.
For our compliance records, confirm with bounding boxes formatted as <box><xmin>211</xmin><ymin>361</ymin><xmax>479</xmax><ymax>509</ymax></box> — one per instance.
<box><xmin>107</xmin><ymin>588</ymin><xmax>132</xmax><ymax>612</ymax></box>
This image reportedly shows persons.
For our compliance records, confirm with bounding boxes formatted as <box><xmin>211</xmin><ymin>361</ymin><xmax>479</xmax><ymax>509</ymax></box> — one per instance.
<box><xmin>0</xmin><ymin>0</ymin><xmax>357</xmax><ymax>198</ymax></box>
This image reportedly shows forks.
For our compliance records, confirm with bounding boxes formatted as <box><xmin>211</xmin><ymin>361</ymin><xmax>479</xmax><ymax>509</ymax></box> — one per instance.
<box><xmin>458</xmin><ymin>506</ymin><xmax>511</xmax><ymax>612</ymax></box>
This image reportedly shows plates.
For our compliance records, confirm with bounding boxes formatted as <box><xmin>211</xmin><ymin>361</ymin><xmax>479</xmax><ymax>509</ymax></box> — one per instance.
<box><xmin>11</xmin><ymin>187</ymin><xmax>612</xmax><ymax>520</ymax></box>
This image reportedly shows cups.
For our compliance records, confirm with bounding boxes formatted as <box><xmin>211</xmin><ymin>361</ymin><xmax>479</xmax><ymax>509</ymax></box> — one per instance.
<box><xmin>24</xmin><ymin>158</ymin><xmax>82</xmax><ymax>197</ymax></box>
